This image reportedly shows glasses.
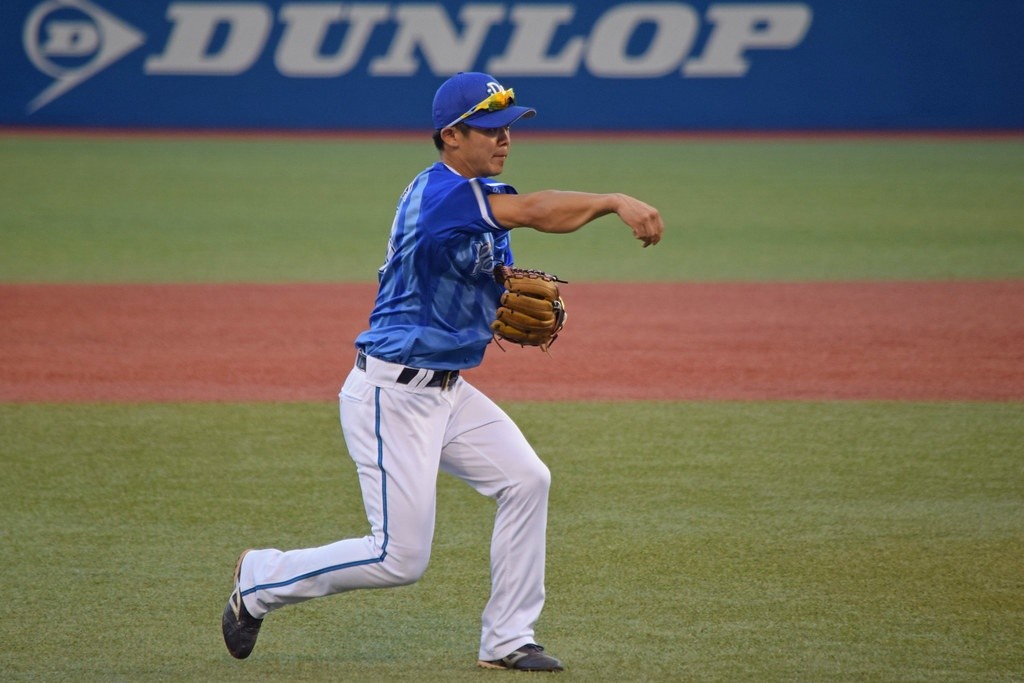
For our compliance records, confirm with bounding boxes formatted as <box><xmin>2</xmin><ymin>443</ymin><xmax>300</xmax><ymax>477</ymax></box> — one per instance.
<box><xmin>446</xmin><ymin>88</ymin><xmax>516</xmax><ymax>129</ymax></box>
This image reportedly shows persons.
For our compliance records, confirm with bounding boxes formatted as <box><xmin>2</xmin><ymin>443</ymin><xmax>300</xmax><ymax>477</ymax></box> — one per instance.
<box><xmin>222</xmin><ymin>72</ymin><xmax>663</xmax><ymax>672</ymax></box>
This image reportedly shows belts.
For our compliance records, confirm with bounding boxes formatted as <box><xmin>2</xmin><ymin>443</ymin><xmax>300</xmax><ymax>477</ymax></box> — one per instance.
<box><xmin>357</xmin><ymin>352</ymin><xmax>459</xmax><ymax>391</ymax></box>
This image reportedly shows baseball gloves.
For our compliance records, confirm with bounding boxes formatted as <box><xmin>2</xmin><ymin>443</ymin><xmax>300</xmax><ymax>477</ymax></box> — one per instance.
<box><xmin>488</xmin><ymin>257</ymin><xmax>571</xmax><ymax>360</ymax></box>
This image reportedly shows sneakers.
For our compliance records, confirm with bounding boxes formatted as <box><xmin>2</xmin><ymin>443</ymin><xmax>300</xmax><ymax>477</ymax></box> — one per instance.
<box><xmin>221</xmin><ymin>549</ymin><xmax>264</xmax><ymax>660</ymax></box>
<box><xmin>477</xmin><ymin>644</ymin><xmax>564</xmax><ymax>672</ymax></box>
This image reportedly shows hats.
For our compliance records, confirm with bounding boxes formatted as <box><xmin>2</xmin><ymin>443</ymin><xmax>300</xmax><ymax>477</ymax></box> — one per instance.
<box><xmin>430</xmin><ymin>72</ymin><xmax>536</xmax><ymax>132</ymax></box>
